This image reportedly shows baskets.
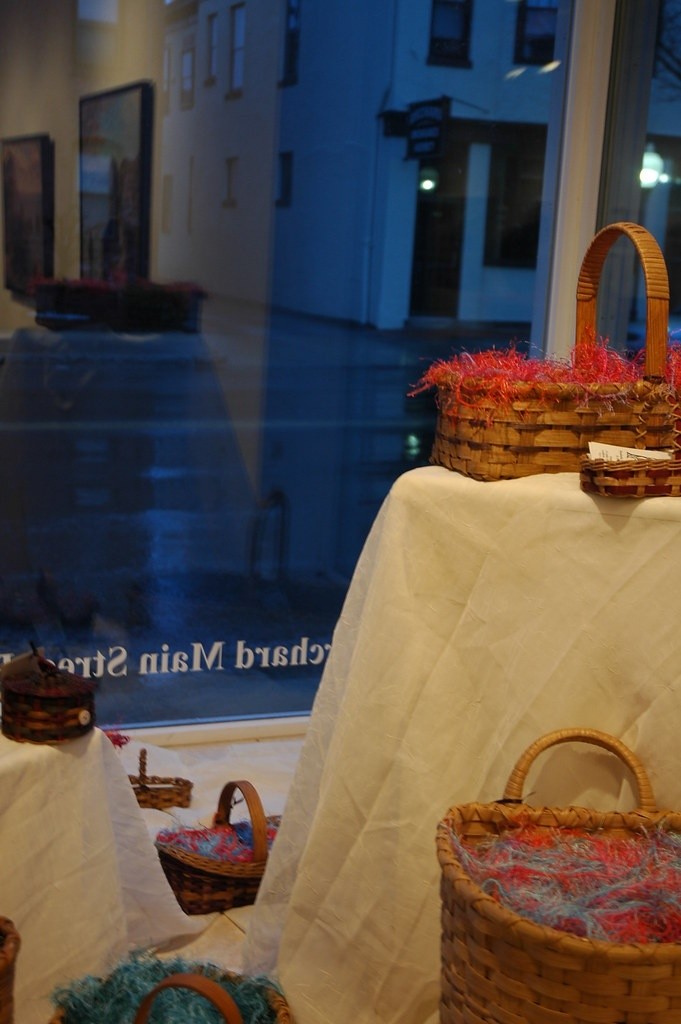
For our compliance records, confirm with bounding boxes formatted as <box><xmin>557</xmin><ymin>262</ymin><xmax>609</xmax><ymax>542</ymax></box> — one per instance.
<box><xmin>431</xmin><ymin>222</ymin><xmax>681</xmax><ymax>496</ymax></box>
<box><xmin>435</xmin><ymin>728</ymin><xmax>681</xmax><ymax>1024</ymax></box>
<box><xmin>0</xmin><ymin>916</ymin><xmax>21</xmax><ymax>1024</ymax></box>
<box><xmin>2</xmin><ymin>669</ymin><xmax>95</xmax><ymax>744</ymax></box>
<box><xmin>154</xmin><ymin>781</ymin><xmax>282</xmax><ymax>916</ymax></box>
<box><xmin>127</xmin><ymin>748</ymin><xmax>193</xmax><ymax>810</ymax></box>
<box><xmin>48</xmin><ymin>962</ymin><xmax>293</xmax><ymax>1024</ymax></box>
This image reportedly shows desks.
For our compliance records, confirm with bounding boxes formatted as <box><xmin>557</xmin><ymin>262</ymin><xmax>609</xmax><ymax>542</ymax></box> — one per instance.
<box><xmin>0</xmin><ymin>726</ymin><xmax>206</xmax><ymax>1024</ymax></box>
<box><xmin>248</xmin><ymin>462</ymin><xmax>681</xmax><ymax>1024</ymax></box>
